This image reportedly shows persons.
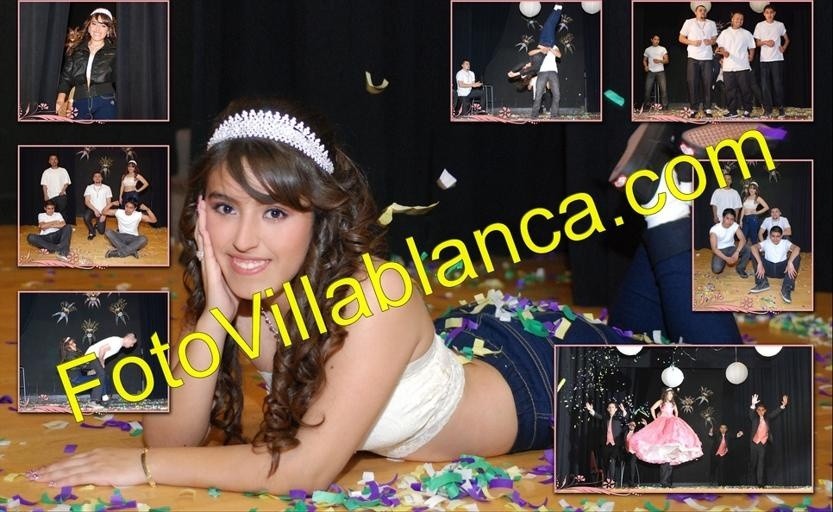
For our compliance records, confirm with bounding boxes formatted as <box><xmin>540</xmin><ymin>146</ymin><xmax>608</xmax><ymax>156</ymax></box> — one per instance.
<box><xmin>625</xmin><ymin>388</ymin><xmax>703</xmax><ymax>466</ymax></box>
<box><xmin>585</xmin><ymin>401</ymin><xmax>629</xmax><ymax>487</ymax></box>
<box><xmin>455</xmin><ymin>60</ymin><xmax>486</xmax><ymax>116</ymax></box>
<box><xmin>508</xmin><ymin>2</ymin><xmax>576</xmax><ymax>122</ymax></box>
<box><xmin>40</xmin><ymin>153</ymin><xmax>72</xmax><ymax>226</ymax></box>
<box><xmin>24</xmin><ymin>98</ymin><xmax>782</xmax><ymax>498</ymax></box>
<box><xmin>83</xmin><ymin>171</ymin><xmax>113</xmax><ymax>240</ymax></box>
<box><xmin>27</xmin><ymin>201</ymin><xmax>73</xmax><ymax>263</ymax></box>
<box><xmin>747</xmin><ymin>393</ymin><xmax>788</xmax><ymax>488</ymax></box>
<box><xmin>101</xmin><ymin>197</ymin><xmax>158</xmax><ymax>260</ymax></box>
<box><xmin>624</xmin><ymin>418</ymin><xmax>648</xmax><ymax>488</ymax></box>
<box><xmin>54</xmin><ymin>7</ymin><xmax>117</xmax><ymax>120</ymax></box>
<box><xmin>118</xmin><ymin>160</ymin><xmax>149</xmax><ymax>209</ymax></box>
<box><xmin>678</xmin><ymin>5</ymin><xmax>790</xmax><ymax>119</ymax></box>
<box><xmin>59</xmin><ymin>336</ymin><xmax>106</xmax><ymax>410</ymax></box>
<box><xmin>642</xmin><ymin>33</ymin><xmax>669</xmax><ymax>114</ymax></box>
<box><xmin>708</xmin><ymin>424</ymin><xmax>743</xmax><ymax>486</ymax></box>
<box><xmin>84</xmin><ymin>332</ymin><xmax>138</xmax><ymax>401</ymax></box>
<box><xmin>709</xmin><ymin>173</ymin><xmax>801</xmax><ymax>304</ymax></box>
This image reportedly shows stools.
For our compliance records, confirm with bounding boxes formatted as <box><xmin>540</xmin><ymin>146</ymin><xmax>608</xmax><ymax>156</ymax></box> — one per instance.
<box><xmin>647</xmin><ymin>80</ymin><xmax>663</xmax><ymax>106</ymax></box>
<box><xmin>470</xmin><ymin>81</ymin><xmax>493</xmax><ymax>114</ymax></box>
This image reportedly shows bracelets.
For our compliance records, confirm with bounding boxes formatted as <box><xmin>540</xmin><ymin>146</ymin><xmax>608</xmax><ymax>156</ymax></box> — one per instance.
<box><xmin>140</xmin><ymin>447</ymin><xmax>156</xmax><ymax>488</ymax></box>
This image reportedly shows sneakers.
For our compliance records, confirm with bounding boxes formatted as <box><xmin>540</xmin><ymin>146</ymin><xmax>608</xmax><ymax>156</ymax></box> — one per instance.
<box><xmin>751</xmin><ymin>280</ymin><xmax>769</xmax><ymax>292</ymax></box>
<box><xmin>106</xmin><ymin>249</ymin><xmax>112</xmax><ymax>258</ymax></box>
<box><xmin>738</xmin><ymin>270</ymin><xmax>748</xmax><ymax>277</ymax></box>
<box><xmin>691</xmin><ymin>111</ymin><xmax>787</xmax><ymax>119</ymax></box>
<box><xmin>88</xmin><ymin>234</ymin><xmax>95</xmax><ymax>239</ymax></box>
<box><xmin>57</xmin><ymin>255</ymin><xmax>68</xmax><ymax>262</ymax></box>
<box><xmin>88</xmin><ymin>395</ymin><xmax>109</xmax><ymax>409</ymax></box>
<box><xmin>780</xmin><ymin>288</ymin><xmax>791</xmax><ymax>302</ymax></box>
<box><xmin>133</xmin><ymin>251</ymin><xmax>138</xmax><ymax>258</ymax></box>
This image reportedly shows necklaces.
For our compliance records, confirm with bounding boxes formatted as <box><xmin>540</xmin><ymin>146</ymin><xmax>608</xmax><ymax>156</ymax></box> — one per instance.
<box><xmin>258</xmin><ymin>303</ymin><xmax>279</xmax><ymax>340</ymax></box>
